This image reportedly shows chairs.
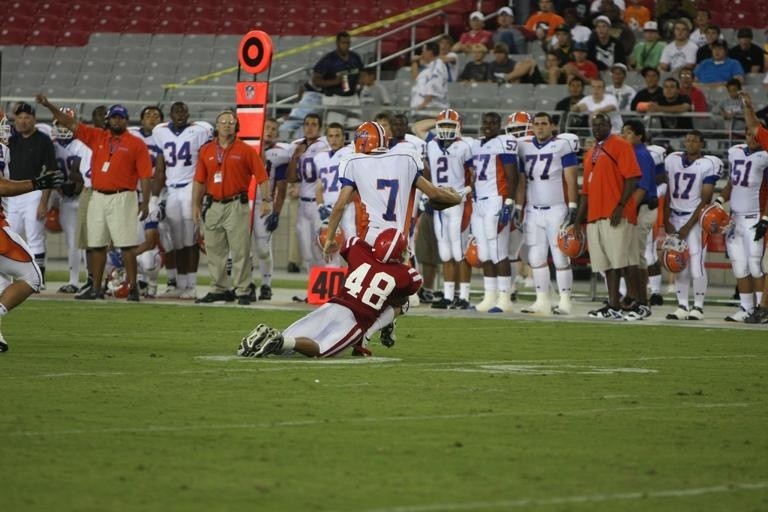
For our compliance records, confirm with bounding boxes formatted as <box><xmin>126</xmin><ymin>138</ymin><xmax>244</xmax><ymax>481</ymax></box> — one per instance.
<box><xmin>0</xmin><ymin>0</ymin><xmax>768</xmax><ymax>129</ymax></box>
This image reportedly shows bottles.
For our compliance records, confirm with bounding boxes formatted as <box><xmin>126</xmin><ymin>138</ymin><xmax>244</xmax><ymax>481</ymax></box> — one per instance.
<box><xmin>339</xmin><ymin>72</ymin><xmax>350</xmax><ymax>92</ymax></box>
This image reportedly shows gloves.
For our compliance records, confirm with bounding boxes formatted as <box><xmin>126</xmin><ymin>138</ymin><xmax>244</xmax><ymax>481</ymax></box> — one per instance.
<box><xmin>264</xmin><ymin>211</ymin><xmax>279</xmax><ymax>232</ymax></box>
<box><xmin>563</xmin><ymin>202</ymin><xmax>577</xmax><ymax>223</ymax></box>
<box><xmin>32</xmin><ymin>165</ymin><xmax>65</xmax><ymax>189</ymax></box>
<box><xmin>752</xmin><ymin>214</ymin><xmax>768</xmax><ymax>241</ymax></box>
<box><xmin>495</xmin><ymin>197</ymin><xmax>523</xmax><ymax>233</ymax></box>
<box><xmin>148</xmin><ymin>195</ymin><xmax>162</xmax><ymax>221</ymax></box>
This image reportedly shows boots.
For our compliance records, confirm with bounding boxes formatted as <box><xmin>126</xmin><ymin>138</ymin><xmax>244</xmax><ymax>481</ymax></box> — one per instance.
<box><xmin>552</xmin><ymin>292</ymin><xmax>571</xmax><ymax>315</ymax></box>
<box><xmin>469</xmin><ymin>290</ymin><xmax>497</xmax><ymax>312</ymax></box>
<box><xmin>488</xmin><ymin>292</ymin><xmax>514</xmax><ymax>313</ymax></box>
<box><xmin>521</xmin><ymin>287</ymin><xmax>553</xmax><ymax>314</ymax></box>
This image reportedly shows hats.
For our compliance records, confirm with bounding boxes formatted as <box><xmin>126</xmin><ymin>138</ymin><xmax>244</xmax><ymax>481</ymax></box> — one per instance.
<box><xmin>108</xmin><ymin>105</ymin><xmax>129</xmax><ymax>120</ymax></box>
<box><xmin>468</xmin><ymin>7</ymin><xmax>748</xmax><ymax>76</ymax></box>
<box><xmin>15</xmin><ymin>102</ymin><xmax>37</xmax><ymax>116</ymax></box>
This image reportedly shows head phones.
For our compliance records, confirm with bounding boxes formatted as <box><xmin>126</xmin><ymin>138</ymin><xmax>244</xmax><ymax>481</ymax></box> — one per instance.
<box><xmin>213</xmin><ymin>110</ymin><xmax>240</xmax><ymax>138</ymax></box>
<box><xmin>101</xmin><ymin>110</ymin><xmax>110</xmax><ymax>131</ymax></box>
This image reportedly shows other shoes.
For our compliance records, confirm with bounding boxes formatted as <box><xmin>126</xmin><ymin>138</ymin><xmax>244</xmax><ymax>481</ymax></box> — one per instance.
<box><xmin>510</xmin><ymin>292</ymin><xmax>516</xmax><ymax>303</ymax></box>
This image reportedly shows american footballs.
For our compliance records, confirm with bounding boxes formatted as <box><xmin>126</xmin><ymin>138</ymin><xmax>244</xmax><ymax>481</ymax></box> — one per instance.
<box><xmin>424</xmin><ymin>187</ymin><xmax>458</xmax><ymax>210</ymax></box>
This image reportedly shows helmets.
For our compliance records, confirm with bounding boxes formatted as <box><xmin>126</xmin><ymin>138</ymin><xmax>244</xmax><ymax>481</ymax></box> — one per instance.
<box><xmin>0</xmin><ymin>112</ymin><xmax>11</xmax><ymax>146</ymax></box>
<box><xmin>464</xmin><ymin>236</ymin><xmax>481</xmax><ymax>268</ymax></box>
<box><xmin>372</xmin><ymin>229</ymin><xmax>408</xmax><ymax>265</ymax></box>
<box><xmin>353</xmin><ymin>120</ymin><xmax>387</xmax><ymax>154</ymax></box>
<box><xmin>659</xmin><ymin>233</ymin><xmax>689</xmax><ymax>274</ymax></box>
<box><xmin>504</xmin><ymin>111</ymin><xmax>533</xmax><ymax>138</ymax></box>
<box><xmin>435</xmin><ymin>108</ymin><xmax>462</xmax><ymax>141</ymax></box>
<box><xmin>316</xmin><ymin>217</ymin><xmax>345</xmax><ymax>253</ymax></box>
<box><xmin>698</xmin><ymin>202</ymin><xmax>732</xmax><ymax>236</ymax></box>
<box><xmin>52</xmin><ymin>107</ymin><xmax>75</xmax><ymax>140</ymax></box>
<box><xmin>558</xmin><ymin>223</ymin><xmax>587</xmax><ymax>260</ymax></box>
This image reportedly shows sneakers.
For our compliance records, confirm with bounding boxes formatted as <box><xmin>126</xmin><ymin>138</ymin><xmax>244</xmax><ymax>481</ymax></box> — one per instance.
<box><xmin>0</xmin><ymin>331</ymin><xmax>9</xmax><ymax>353</ymax></box>
<box><xmin>724</xmin><ymin>306</ymin><xmax>754</xmax><ymax>321</ymax></box>
<box><xmin>59</xmin><ymin>279</ymin><xmax>272</xmax><ymax>305</ymax></box>
<box><xmin>620</xmin><ymin>296</ymin><xmax>636</xmax><ymax>308</ymax></box>
<box><xmin>248</xmin><ymin>327</ymin><xmax>284</xmax><ymax>358</ymax></box>
<box><xmin>288</xmin><ymin>263</ymin><xmax>300</xmax><ymax>272</ymax></box>
<box><xmin>666</xmin><ymin>306</ymin><xmax>690</xmax><ymax>320</ymax></box>
<box><xmin>380</xmin><ymin>319</ymin><xmax>396</xmax><ymax>349</ymax></box>
<box><xmin>450</xmin><ymin>299</ymin><xmax>469</xmax><ymax>309</ymax></box>
<box><xmin>418</xmin><ymin>288</ymin><xmax>444</xmax><ymax>302</ymax></box>
<box><xmin>688</xmin><ymin>306</ymin><xmax>704</xmax><ymax>320</ymax></box>
<box><xmin>625</xmin><ymin>304</ymin><xmax>651</xmax><ymax>321</ymax></box>
<box><xmin>648</xmin><ymin>293</ymin><xmax>664</xmax><ymax>306</ymax></box>
<box><xmin>588</xmin><ymin>301</ymin><xmax>624</xmax><ymax>320</ymax></box>
<box><xmin>236</xmin><ymin>323</ymin><xmax>268</xmax><ymax>356</ymax></box>
<box><xmin>744</xmin><ymin>304</ymin><xmax>766</xmax><ymax>322</ymax></box>
<box><xmin>430</xmin><ymin>299</ymin><xmax>451</xmax><ymax>309</ymax></box>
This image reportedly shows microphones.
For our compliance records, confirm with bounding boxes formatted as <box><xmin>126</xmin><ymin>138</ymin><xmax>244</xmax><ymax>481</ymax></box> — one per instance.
<box><xmin>111</xmin><ymin>124</ymin><xmax>121</xmax><ymax>133</ymax></box>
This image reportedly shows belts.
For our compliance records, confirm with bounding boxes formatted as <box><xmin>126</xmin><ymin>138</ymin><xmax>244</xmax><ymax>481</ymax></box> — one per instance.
<box><xmin>301</xmin><ymin>197</ymin><xmax>315</xmax><ymax>202</ymax></box>
<box><xmin>174</xmin><ymin>183</ymin><xmax>188</xmax><ymax>188</ymax></box>
<box><xmin>98</xmin><ymin>188</ymin><xmax>127</xmax><ymax>194</ymax></box>
<box><xmin>533</xmin><ymin>206</ymin><xmax>550</xmax><ymax>210</ymax></box>
<box><xmin>213</xmin><ymin>196</ymin><xmax>238</xmax><ymax>203</ymax></box>
<box><xmin>673</xmin><ymin>211</ymin><xmax>690</xmax><ymax>216</ymax></box>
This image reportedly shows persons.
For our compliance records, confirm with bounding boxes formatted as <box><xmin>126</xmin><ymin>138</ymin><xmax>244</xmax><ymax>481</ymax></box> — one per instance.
<box><xmin>2</xmin><ymin>92</ymin><xmax>289</xmax><ymax>306</ymax></box>
<box><xmin>237</xmin><ymin>229</ymin><xmax>423</xmax><ymax>357</ymax></box>
<box><xmin>323</xmin><ymin>121</ymin><xmax>462</xmax><ymax>356</ymax></box>
<box><xmin>1</xmin><ymin>114</ymin><xmax>63</xmax><ymax>351</ymax></box>
<box><xmin>263</xmin><ymin>0</ymin><xmax>768</xmax><ymax>323</ymax></box>
